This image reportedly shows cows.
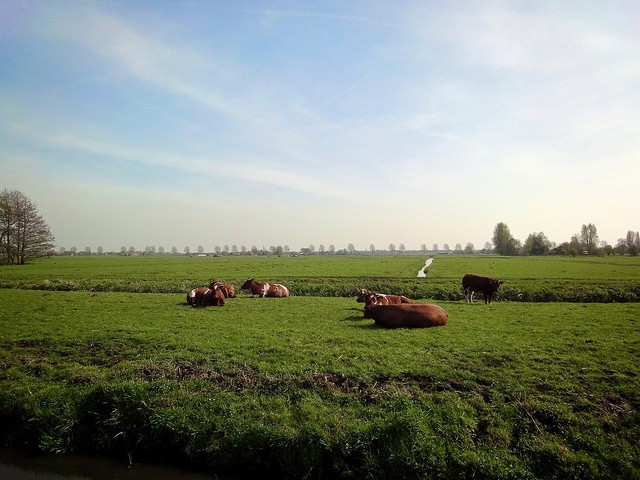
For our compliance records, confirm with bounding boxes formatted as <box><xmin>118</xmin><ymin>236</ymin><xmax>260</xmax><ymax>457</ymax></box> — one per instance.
<box><xmin>364</xmin><ymin>302</ymin><xmax>450</xmax><ymax>328</ymax></box>
<box><xmin>462</xmin><ymin>273</ymin><xmax>505</xmax><ymax>305</ymax></box>
<box><xmin>241</xmin><ymin>277</ymin><xmax>290</xmax><ymax>297</ymax></box>
<box><xmin>210</xmin><ymin>280</ymin><xmax>235</xmax><ymax>298</ymax></box>
<box><xmin>187</xmin><ymin>285</ymin><xmax>225</xmax><ymax>307</ymax></box>
<box><xmin>356</xmin><ymin>289</ymin><xmax>415</xmax><ymax>307</ymax></box>
